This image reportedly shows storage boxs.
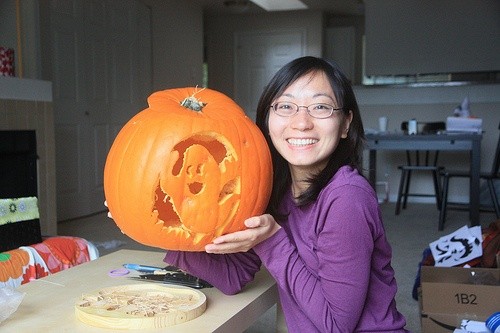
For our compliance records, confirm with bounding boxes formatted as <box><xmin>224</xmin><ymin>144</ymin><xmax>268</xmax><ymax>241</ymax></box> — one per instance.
<box><xmin>418</xmin><ymin>251</ymin><xmax>500</xmax><ymax>333</ymax></box>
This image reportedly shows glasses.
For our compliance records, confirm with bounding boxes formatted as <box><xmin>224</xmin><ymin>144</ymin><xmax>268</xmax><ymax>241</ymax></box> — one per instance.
<box><xmin>269</xmin><ymin>101</ymin><xmax>341</xmax><ymax>119</ymax></box>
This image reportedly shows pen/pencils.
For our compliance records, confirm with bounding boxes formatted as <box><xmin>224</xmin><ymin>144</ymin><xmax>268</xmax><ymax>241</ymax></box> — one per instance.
<box><xmin>122</xmin><ymin>264</ymin><xmax>166</xmax><ymax>272</ymax></box>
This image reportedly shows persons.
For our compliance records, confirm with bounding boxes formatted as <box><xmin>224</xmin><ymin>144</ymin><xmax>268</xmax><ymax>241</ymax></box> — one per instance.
<box><xmin>104</xmin><ymin>56</ymin><xmax>411</xmax><ymax>333</ymax></box>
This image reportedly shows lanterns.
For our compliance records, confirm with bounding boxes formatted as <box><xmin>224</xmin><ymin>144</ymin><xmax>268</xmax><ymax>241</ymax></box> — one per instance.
<box><xmin>102</xmin><ymin>87</ymin><xmax>274</xmax><ymax>252</ymax></box>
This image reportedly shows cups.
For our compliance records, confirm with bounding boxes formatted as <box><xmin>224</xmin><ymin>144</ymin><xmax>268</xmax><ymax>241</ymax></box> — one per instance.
<box><xmin>379</xmin><ymin>116</ymin><xmax>388</xmax><ymax>131</ymax></box>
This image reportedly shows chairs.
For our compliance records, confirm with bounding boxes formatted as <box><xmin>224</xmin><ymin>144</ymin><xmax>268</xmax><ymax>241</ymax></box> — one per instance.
<box><xmin>394</xmin><ymin>120</ymin><xmax>445</xmax><ymax>215</ymax></box>
<box><xmin>438</xmin><ymin>122</ymin><xmax>500</xmax><ymax>231</ymax></box>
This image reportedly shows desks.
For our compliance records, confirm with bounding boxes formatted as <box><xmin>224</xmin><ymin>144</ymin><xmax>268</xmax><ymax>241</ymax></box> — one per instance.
<box><xmin>363</xmin><ymin>133</ymin><xmax>483</xmax><ymax>227</ymax></box>
<box><xmin>0</xmin><ymin>249</ymin><xmax>289</xmax><ymax>332</ymax></box>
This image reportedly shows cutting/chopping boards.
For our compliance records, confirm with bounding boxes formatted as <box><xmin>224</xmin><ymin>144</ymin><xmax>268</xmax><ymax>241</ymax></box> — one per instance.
<box><xmin>74</xmin><ymin>283</ymin><xmax>207</xmax><ymax>331</ymax></box>
